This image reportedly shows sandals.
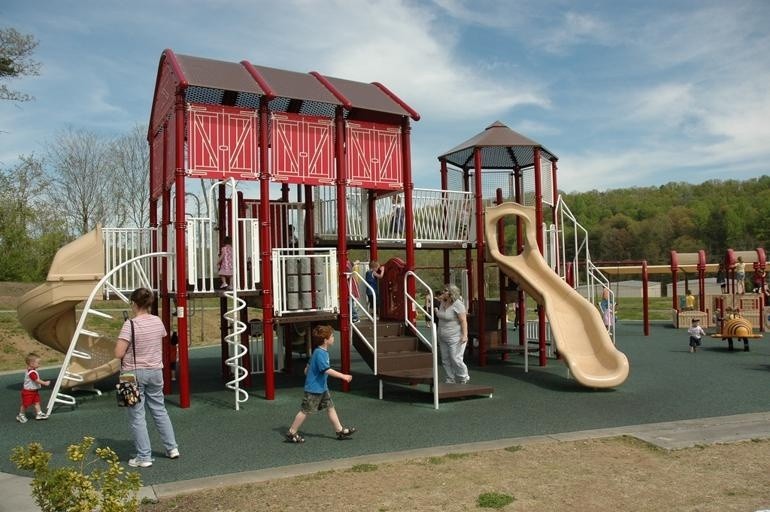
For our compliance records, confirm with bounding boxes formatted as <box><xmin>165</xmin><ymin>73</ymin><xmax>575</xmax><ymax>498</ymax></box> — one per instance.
<box><xmin>285</xmin><ymin>430</ymin><xmax>305</xmax><ymax>443</ymax></box>
<box><xmin>336</xmin><ymin>427</ymin><xmax>357</xmax><ymax>439</ymax></box>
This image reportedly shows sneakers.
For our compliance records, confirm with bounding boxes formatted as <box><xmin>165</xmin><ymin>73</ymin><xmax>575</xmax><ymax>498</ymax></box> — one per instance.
<box><xmin>16</xmin><ymin>413</ymin><xmax>28</xmax><ymax>423</ymax></box>
<box><xmin>461</xmin><ymin>376</ymin><xmax>471</xmax><ymax>385</ymax></box>
<box><xmin>220</xmin><ymin>284</ymin><xmax>233</xmax><ymax>290</ymax></box>
<box><xmin>128</xmin><ymin>457</ymin><xmax>152</xmax><ymax>468</ymax></box>
<box><xmin>353</xmin><ymin>319</ymin><xmax>360</xmax><ymax>324</ymax></box>
<box><xmin>35</xmin><ymin>412</ymin><xmax>49</xmax><ymax>420</ymax></box>
<box><xmin>165</xmin><ymin>448</ymin><xmax>179</xmax><ymax>459</ymax></box>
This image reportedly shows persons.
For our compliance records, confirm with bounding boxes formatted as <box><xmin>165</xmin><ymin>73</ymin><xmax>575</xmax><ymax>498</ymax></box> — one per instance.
<box><xmin>601</xmin><ymin>288</ymin><xmax>617</xmax><ymax>335</ymax></box>
<box><xmin>170</xmin><ymin>330</ymin><xmax>176</xmax><ymax>381</ymax></box>
<box><xmin>285</xmin><ymin>324</ymin><xmax>356</xmax><ymax>443</ymax></box>
<box><xmin>15</xmin><ymin>352</ymin><xmax>51</xmax><ymax>424</ymax></box>
<box><xmin>114</xmin><ymin>287</ymin><xmax>180</xmax><ymax>468</ymax></box>
<box><xmin>347</xmin><ymin>194</ymin><xmax>472</xmax><ymax>330</ymax></box>
<box><xmin>437</xmin><ymin>283</ymin><xmax>470</xmax><ymax>385</ymax></box>
<box><xmin>288</xmin><ymin>224</ymin><xmax>298</xmax><ymax>244</ymax></box>
<box><xmin>679</xmin><ymin>255</ymin><xmax>770</xmax><ymax>353</ymax></box>
<box><xmin>512</xmin><ymin>302</ymin><xmax>519</xmax><ymax>331</ymax></box>
<box><xmin>216</xmin><ymin>237</ymin><xmax>233</xmax><ymax>291</ymax></box>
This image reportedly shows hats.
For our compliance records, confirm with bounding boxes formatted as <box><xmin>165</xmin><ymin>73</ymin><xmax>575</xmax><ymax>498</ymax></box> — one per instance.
<box><xmin>691</xmin><ymin>317</ymin><xmax>700</xmax><ymax>322</ymax></box>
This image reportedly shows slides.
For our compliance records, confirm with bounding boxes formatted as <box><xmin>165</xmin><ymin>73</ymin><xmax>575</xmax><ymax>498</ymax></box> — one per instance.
<box><xmin>16</xmin><ymin>226</ymin><xmax>124</xmax><ymax>390</ymax></box>
<box><xmin>484</xmin><ymin>202</ymin><xmax>629</xmax><ymax>388</ymax></box>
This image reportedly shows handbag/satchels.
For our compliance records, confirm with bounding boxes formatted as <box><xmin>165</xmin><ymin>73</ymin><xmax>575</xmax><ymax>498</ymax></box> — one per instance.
<box><xmin>115</xmin><ymin>381</ymin><xmax>141</xmax><ymax>408</ymax></box>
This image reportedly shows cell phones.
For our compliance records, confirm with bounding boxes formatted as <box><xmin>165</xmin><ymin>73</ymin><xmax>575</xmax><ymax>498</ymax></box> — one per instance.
<box><xmin>123</xmin><ymin>310</ymin><xmax>130</xmax><ymax>321</ymax></box>
